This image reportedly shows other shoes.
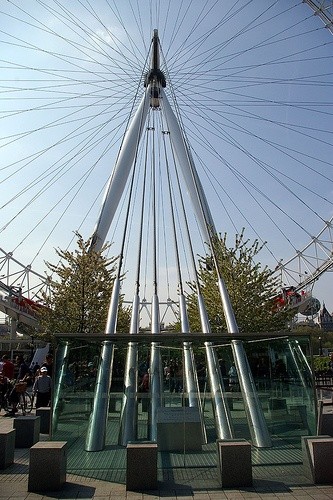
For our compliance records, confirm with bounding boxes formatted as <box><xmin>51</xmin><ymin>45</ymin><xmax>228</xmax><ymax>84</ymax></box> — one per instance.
<box><xmin>4</xmin><ymin>408</ymin><xmax>19</xmax><ymax>417</ymax></box>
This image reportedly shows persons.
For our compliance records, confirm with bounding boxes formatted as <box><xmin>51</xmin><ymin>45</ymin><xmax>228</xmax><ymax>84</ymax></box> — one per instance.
<box><xmin>42</xmin><ymin>354</ymin><xmax>53</xmax><ymax>378</ymax></box>
<box><xmin>1</xmin><ymin>355</ymin><xmax>15</xmax><ymax>380</ymax></box>
<box><xmin>3</xmin><ymin>355</ymin><xmax>32</xmax><ymax>417</ymax></box>
<box><xmin>32</xmin><ymin>366</ymin><xmax>52</xmax><ymax>408</ymax></box>
<box><xmin>70</xmin><ymin>355</ymin><xmax>287</xmax><ymax>391</ymax></box>
<box><xmin>33</xmin><ymin>362</ymin><xmax>40</xmax><ymax>376</ymax></box>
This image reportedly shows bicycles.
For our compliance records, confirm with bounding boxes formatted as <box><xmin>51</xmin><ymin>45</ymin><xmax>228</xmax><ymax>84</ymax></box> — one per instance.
<box><xmin>1</xmin><ymin>377</ymin><xmax>32</xmax><ymax>415</ymax></box>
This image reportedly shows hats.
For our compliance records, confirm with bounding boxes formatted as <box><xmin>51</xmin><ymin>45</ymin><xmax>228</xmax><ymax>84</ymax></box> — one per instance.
<box><xmin>40</xmin><ymin>367</ymin><xmax>48</xmax><ymax>372</ymax></box>
